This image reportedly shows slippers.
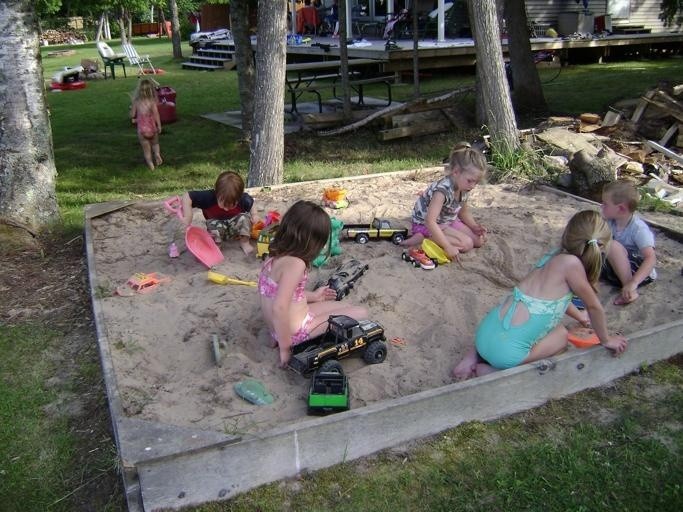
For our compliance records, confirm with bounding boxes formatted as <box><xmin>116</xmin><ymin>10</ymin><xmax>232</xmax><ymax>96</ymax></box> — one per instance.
<box><xmin>234</xmin><ymin>379</ymin><xmax>273</xmax><ymax>406</ymax></box>
<box><xmin>212</xmin><ymin>334</ymin><xmax>228</xmax><ymax>365</ymax></box>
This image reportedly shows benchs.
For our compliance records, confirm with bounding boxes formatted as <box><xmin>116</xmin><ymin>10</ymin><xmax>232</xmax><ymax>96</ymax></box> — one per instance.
<box><xmin>288</xmin><ymin>76</ymin><xmax>395</xmax><ymax>114</ymax></box>
<box><xmin>285</xmin><ymin>72</ymin><xmax>360</xmax><ymax>113</ymax></box>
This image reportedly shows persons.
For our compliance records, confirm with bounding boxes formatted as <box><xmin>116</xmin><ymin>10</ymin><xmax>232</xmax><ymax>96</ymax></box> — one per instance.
<box><xmin>398</xmin><ymin>148</ymin><xmax>487</xmax><ymax>262</ymax></box>
<box><xmin>257</xmin><ymin>200</ymin><xmax>367</xmax><ymax>368</ymax></box>
<box><xmin>599</xmin><ymin>179</ymin><xmax>657</xmax><ymax>305</ymax></box>
<box><xmin>176</xmin><ymin>171</ymin><xmax>255</xmax><ymax>255</ymax></box>
<box><xmin>130</xmin><ymin>79</ymin><xmax>163</xmax><ymax>173</ymax></box>
<box><xmin>450</xmin><ymin>209</ymin><xmax>627</xmax><ymax>382</ymax></box>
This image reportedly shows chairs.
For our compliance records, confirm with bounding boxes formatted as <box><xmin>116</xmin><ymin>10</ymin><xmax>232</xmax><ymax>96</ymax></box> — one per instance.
<box><xmin>296</xmin><ymin>0</ymin><xmax>457</xmax><ymax>44</ymax></box>
<box><xmin>97</xmin><ymin>41</ymin><xmax>126</xmax><ymax>80</ymax></box>
<box><xmin>121</xmin><ymin>43</ymin><xmax>156</xmax><ymax>76</ymax></box>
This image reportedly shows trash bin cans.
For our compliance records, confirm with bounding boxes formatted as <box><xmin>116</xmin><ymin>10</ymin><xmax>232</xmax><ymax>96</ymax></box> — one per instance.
<box><xmin>42</xmin><ymin>39</ymin><xmax>48</xmax><ymax>46</ymax></box>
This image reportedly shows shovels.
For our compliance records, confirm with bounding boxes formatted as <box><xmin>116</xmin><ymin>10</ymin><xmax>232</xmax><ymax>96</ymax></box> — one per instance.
<box><xmin>208</xmin><ymin>271</ymin><xmax>257</xmax><ymax>287</ymax></box>
<box><xmin>162</xmin><ymin>195</ymin><xmax>225</xmax><ymax>270</ymax></box>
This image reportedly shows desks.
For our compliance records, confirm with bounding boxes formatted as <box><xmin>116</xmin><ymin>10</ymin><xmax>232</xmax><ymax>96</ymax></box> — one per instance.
<box><xmin>286</xmin><ymin>59</ymin><xmax>388</xmax><ymax>114</ymax></box>
